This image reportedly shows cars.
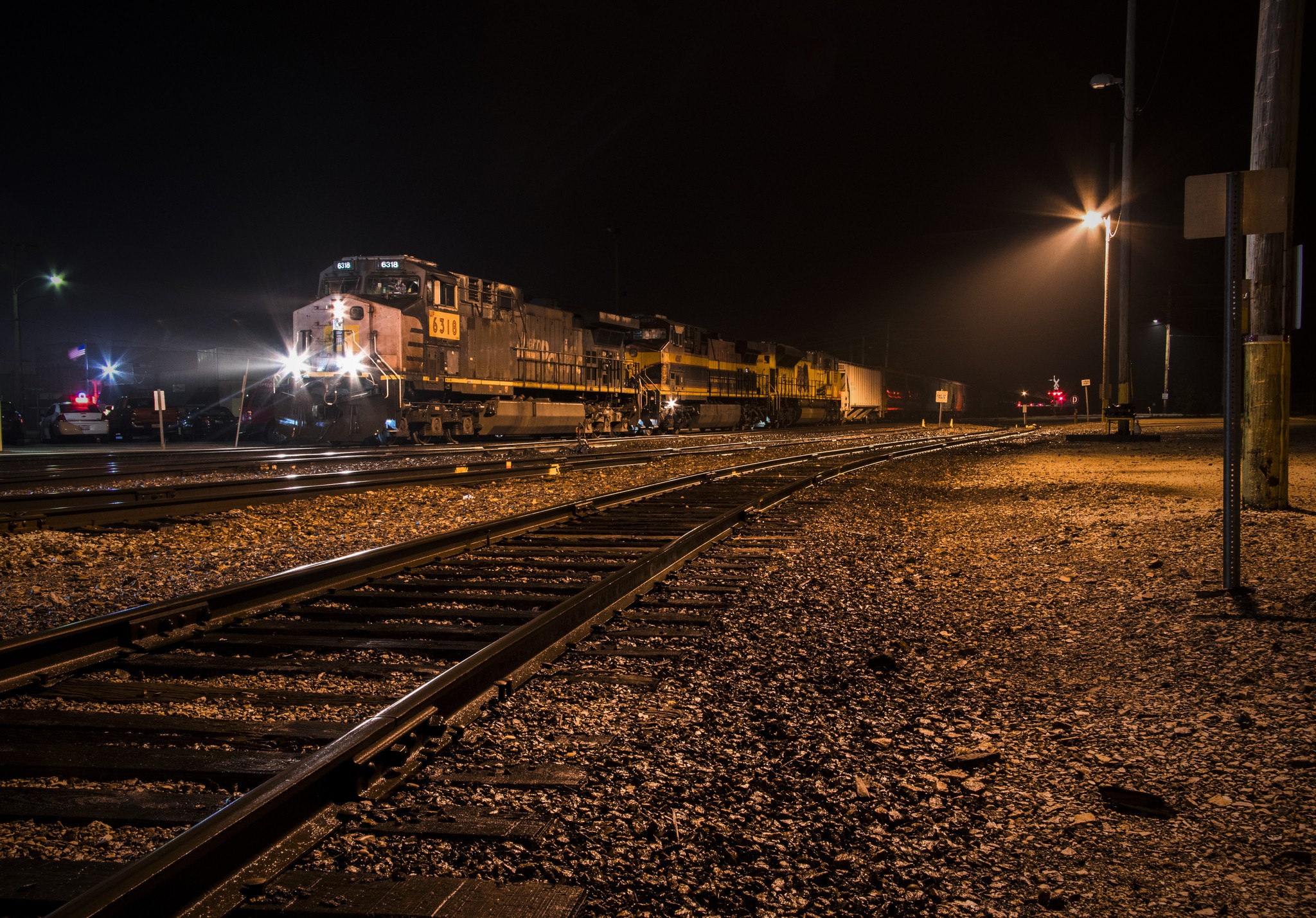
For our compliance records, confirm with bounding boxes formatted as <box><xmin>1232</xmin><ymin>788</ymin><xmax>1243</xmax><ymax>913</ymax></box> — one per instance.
<box><xmin>180</xmin><ymin>406</ymin><xmax>240</xmax><ymax>442</ymax></box>
<box><xmin>39</xmin><ymin>401</ymin><xmax>110</xmax><ymax>444</ymax></box>
<box><xmin>1</xmin><ymin>402</ymin><xmax>25</xmax><ymax>446</ymax></box>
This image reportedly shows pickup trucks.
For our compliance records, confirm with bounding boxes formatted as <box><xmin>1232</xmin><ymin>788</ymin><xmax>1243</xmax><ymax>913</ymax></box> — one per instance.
<box><xmin>106</xmin><ymin>395</ymin><xmax>181</xmax><ymax>442</ymax></box>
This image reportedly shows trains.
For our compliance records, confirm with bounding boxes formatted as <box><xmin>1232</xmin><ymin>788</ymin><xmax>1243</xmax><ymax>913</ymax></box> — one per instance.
<box><xmin>289</xmin><ymin>252</ymin><xmax>970</xmax><ymax>448</ymax></box>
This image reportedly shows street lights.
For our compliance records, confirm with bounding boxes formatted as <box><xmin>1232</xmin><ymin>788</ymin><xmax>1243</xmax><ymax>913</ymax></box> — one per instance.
<box><xmin>1152</xmin><ymin>319</ymin><xmax>1171</xmax><ymax>414</ymax></box>
<box><xmin>1078</xmin><ymin>205</ymin><xmax>1112</xmax><ymax>423</ymax></box>
<box><xmin>1089</xmin><ymin>73</ymin><xmax>1137</xmax><ymax>438</ymax></box>
<box><xmin>13</xmin><ymin>274</ymin><xmax>64</xmax><ymax>415</ymax></box>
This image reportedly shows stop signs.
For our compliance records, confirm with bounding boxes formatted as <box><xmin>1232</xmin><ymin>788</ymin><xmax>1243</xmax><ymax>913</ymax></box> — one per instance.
<box><xmin>260</xmin><ymin>379</ymin><xmax>274</xmax><ymax>388</ymax></box>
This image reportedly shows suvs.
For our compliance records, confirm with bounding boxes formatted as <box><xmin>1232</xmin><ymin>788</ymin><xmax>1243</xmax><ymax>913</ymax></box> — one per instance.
<box><xmin>237</xmin><ymin>379</ymin><xmax>290</xmax><ymax>445</ymax></box>
